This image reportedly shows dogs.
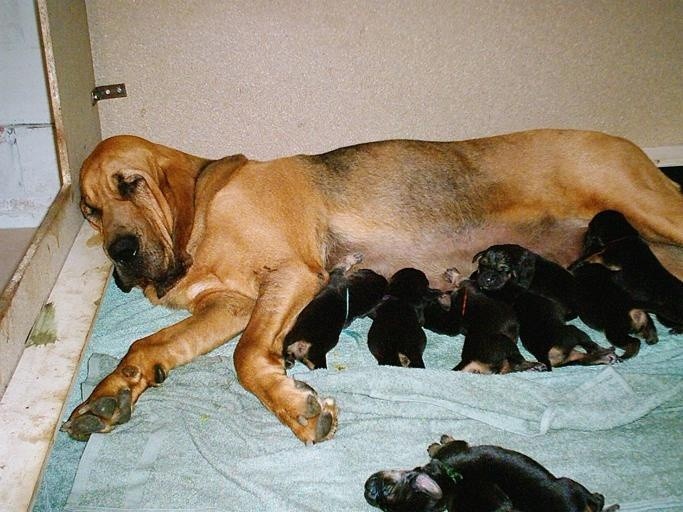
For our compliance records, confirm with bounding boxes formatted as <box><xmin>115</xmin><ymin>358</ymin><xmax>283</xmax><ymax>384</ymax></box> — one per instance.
<box><xmin>367</xmin><ymin>267</ymin><xmax>442</xmax><ymax>370</ymax></box>
<box><xmin>573</xmin><ymin>262</ymin><xmax>660</xmax><ymax>361</ymax></box>
<box><xmin>58</xmin><ymin>127</ymin><xmax>683</xmax><ymax>446</ymax></box>
<box><xmin>364</xmin><ymin>434</ymin><xmax>620</xmax><ymax>512</ymax></box>
<box><xmin>582</xmin><ymin>208</ymin><xmax>683</xmax><ymax>335</ymax></box>
<box><xmin>282</xmin><ymin>268</ymin><xmax>389</xmax><ymax>371</ymax></box>
<box><xmin>472</xmin><ymin>244</ymin><xmax>583</xmax><ymax>321</ymax></box>
<box><xmin>417</xmin><ymin>268</ymin><xmax>624</xmax><ymax>375</ymax></box>
